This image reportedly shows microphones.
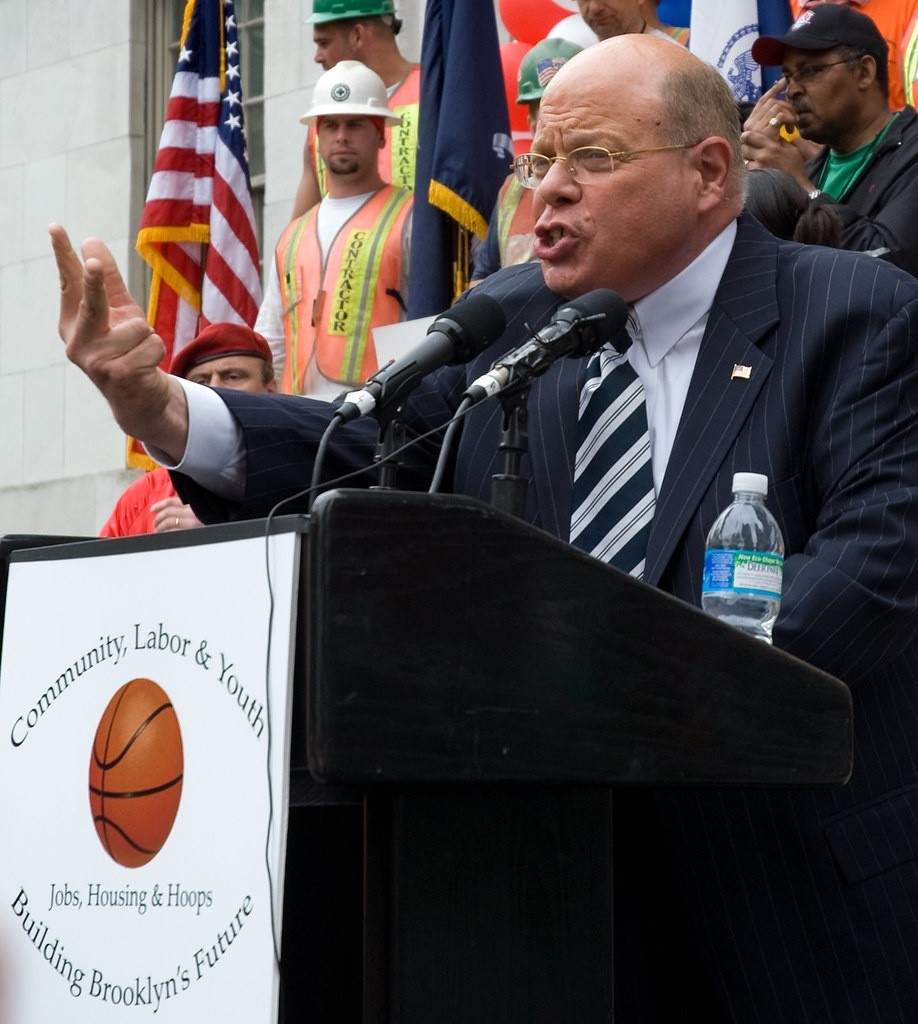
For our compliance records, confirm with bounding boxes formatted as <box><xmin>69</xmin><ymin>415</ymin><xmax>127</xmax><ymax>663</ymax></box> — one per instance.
<box><xmin>465</xmin><ymin>287</ymin><xmax>630</xmax><ymax>406</ymax></box>
<box><xmin>335</xmin><ymin>293</ymin><xmax>506</xmax><ymax>423</ymax></box>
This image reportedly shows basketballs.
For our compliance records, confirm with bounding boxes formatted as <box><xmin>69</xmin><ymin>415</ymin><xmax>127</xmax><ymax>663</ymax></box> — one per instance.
<box><xmin>87</xmin><ymin>677</ymin><xmax>184</xmax><ymax>868</ymax></box>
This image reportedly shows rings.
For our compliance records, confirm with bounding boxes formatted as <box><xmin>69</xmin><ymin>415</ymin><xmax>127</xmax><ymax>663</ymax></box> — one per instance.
<box><xmin>176</xmin><ymin>517</ymin><xmax>179</xmax><ymax>527</ymax></box>
<box><xmin>768</xmin><ymin>118</ymin><xmax>780</xmax><ymax>128</ymax></box>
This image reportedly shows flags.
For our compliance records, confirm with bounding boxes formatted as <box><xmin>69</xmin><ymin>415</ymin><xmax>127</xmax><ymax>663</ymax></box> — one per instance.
<box><xmin>407</xmin><ymin>1</ymin><xmax>514</xmax><ymax>320</ymax></box>
<box><xmin>125</xmin><ymin>0</ymin><xmax>264</xmax><ymax>473</ymax></box>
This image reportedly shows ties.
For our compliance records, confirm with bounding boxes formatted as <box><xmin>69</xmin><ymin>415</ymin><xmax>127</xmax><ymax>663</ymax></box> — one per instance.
<box><xmin>569</xmin><ymin>307</ymin><xmax>656</xmax><ymax>582</ymax></box>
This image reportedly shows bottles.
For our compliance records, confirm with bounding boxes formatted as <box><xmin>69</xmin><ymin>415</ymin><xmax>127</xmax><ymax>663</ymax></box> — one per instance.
<box><xmin>701</xmin><ymin>472</ymin><xmax>784</xmax><ymax>645</ymax></box>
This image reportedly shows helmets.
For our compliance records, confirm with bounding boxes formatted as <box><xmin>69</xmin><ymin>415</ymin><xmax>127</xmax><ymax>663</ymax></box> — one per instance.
<box><xmin>514</xmin><ymin>38</ymin><xmax>584</xmax><ymax>104</ymax></box>
<box><xmin>299</xmin><ymin>60</ymin><xmax>403</xmax><ymax>127</ymax></box>
<box><xmin>305</xmin><ymin>0</ymin><xmax>398</xmax><ymax>24</ymax></box>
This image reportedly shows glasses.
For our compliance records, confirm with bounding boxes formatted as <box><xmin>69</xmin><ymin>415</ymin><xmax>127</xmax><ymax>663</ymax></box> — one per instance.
<box><xmin>510</xmin><ymin>140</ymin><xmax>702</xmax><ymax>190</ymax></box>
<box><xmin>780</xmin><ymin>60</ymin><xmax>850</xmax><ymax>83</ymax></box>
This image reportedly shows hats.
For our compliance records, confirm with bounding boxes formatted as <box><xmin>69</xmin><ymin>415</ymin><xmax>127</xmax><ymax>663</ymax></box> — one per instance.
<box><xmin>169</xmin><ymin>322</ymin><xmax>272</xmax><ymax>378</ymax></box>
<box><xmin>752</xmin><ymin>4</ymin><xmax>888</xmax><ymax>66</ymax></box>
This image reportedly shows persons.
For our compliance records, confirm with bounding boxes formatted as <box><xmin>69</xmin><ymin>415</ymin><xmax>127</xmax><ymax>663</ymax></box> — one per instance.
<box><xmin>47</xmin><ymin>34</ymin><xmax>918</xmax><ymax>1017</ymax></box>
<box><xmin>253</xmin><ymin>61</ymin><xmax>414</xmax><ymax>403</ymax></box>
<box><xmin>470</xmin><ymin>38</ymin><xmax>584</xmax><ymax>291</ymax></box>
<box><xmin>98</xmin><ymin>324</ymin><xmax>278</xmax><ymax>538</ymax></box>
<box><xmin>291</xmin><ymin>1</ymin><xmax>420</xmax><ymax>224</ymax></box>
<box><xmin>579</xmin><ymin>1</ymin><xmax>690</xmax><ymax>47</ymax></box>
<box><xmin>739</xmin><ymin>3</ymin><xmax>918</xmax><ymax>278</ymax></box>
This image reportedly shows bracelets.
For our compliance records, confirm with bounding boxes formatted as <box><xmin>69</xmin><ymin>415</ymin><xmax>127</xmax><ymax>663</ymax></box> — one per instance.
<box><xmin>808</xmin><ymin>189</ymin><xmax>821</xmax><ymax>199</ymax></box>
